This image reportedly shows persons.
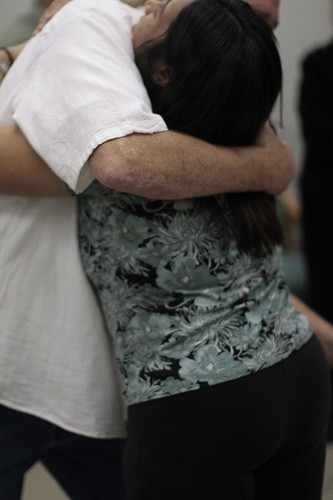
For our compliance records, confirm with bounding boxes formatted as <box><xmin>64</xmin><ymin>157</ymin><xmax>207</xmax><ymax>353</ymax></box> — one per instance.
<box><xmin>1</xmin><ymin>0</ymin><xmax>332</xmax><ymax>499</ymax></box>
